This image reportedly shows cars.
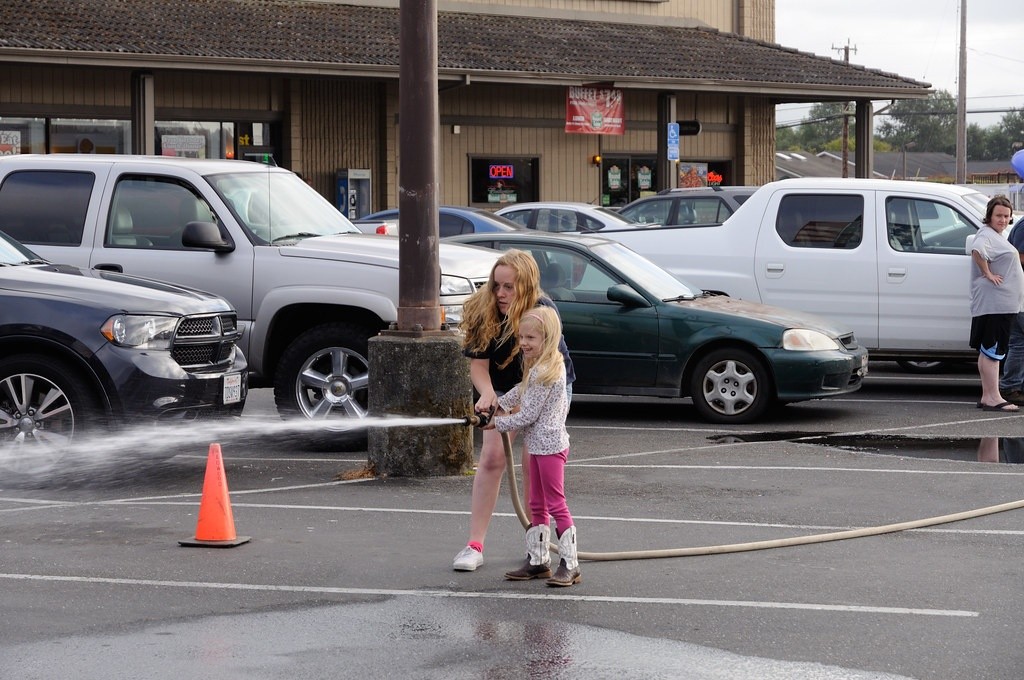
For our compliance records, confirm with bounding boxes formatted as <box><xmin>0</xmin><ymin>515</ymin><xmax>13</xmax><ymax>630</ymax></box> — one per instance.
<box><xmin>361</xmin><ymin>202</ymin><xmax>866</xmax><ymax>425</ymax></box>
<box><xmin>619</xmin><ymin>187</ymin><xmax>762</xmax><ymax>225</ymax></box>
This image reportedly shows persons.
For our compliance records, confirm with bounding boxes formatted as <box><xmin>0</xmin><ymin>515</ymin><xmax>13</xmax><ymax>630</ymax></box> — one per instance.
<box><xmin>478</xmin><ymin>306</ymin><xmax>583</xmax><ymax>586</ymax></box>
<box><xmin>1000</xmin><ymin>216</ymin><xmax>1024</xmax><ymax>406</ymax></box>
<box><xmin>452</xmin><ymin>248</ymin><xmax>574</xmax><ymax>572</ymax></box>
<box><xmin>970</xmin><ymin>196</ymin><xmax>1021</xmax><ymax>413</ymax></box>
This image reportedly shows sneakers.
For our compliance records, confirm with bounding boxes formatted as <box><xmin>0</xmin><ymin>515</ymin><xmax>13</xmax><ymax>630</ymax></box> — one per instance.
<box><xmin>1001</xmin><ymin>392</ymin><xmax>1024</xmax><ymax>405</ymax></box>
<box><xmin>452</xmin><ymin>547</ymin><xmax>484</xmax><ymax>571</ymax></box>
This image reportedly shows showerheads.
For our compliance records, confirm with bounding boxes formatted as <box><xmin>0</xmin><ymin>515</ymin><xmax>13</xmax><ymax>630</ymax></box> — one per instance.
<box><xmin>461</xmin><ymin>413</ymin><xmax>486</xmax><ymax>428</ymax></box>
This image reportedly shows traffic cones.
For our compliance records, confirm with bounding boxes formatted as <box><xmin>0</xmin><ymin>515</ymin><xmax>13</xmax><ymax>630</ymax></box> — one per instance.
<box><xmin>177</xmin><ymin>442</ymin><xmax>252</xmax><ymax>547</ymax></box>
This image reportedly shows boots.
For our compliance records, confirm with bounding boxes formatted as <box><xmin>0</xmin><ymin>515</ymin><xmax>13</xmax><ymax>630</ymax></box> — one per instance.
<box><xmin>504</xmin><ymin>522</ymin><xmax>551</xmax><ymax>580</ymax></box>
<box><xmin>547</xmin><ymin>526</ymin><xmax>581</xmax><ymax>587</ymax></box>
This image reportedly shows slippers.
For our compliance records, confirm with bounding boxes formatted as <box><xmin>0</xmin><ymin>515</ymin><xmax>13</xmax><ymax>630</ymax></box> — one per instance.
<box><xmin>977</xmin><ymin>401</ymin><xmax>984</xmax><ymax>408</ymax></box>
<box><xmin>983</xmin><ymin>402</ymin><xmax>1020</xmax><ymax>412</ymax></box>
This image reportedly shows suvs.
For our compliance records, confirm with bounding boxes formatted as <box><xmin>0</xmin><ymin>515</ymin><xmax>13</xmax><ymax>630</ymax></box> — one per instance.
<box><xmin>1</xmin><ymin>151</ymin><xmax>504</xmax><ymax>454</ymax></box>
<box><xmin>0</xmin><ymin>232</ymin><xmax>249</xmax><ymax>475</ymax></box>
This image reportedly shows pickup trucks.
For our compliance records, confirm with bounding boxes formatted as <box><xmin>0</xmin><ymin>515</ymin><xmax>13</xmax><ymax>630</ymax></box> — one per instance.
<box><xmin>580</xmin><ymin>177</ymin><xmax>1022</xmax><ymax>360</ymax></box>
